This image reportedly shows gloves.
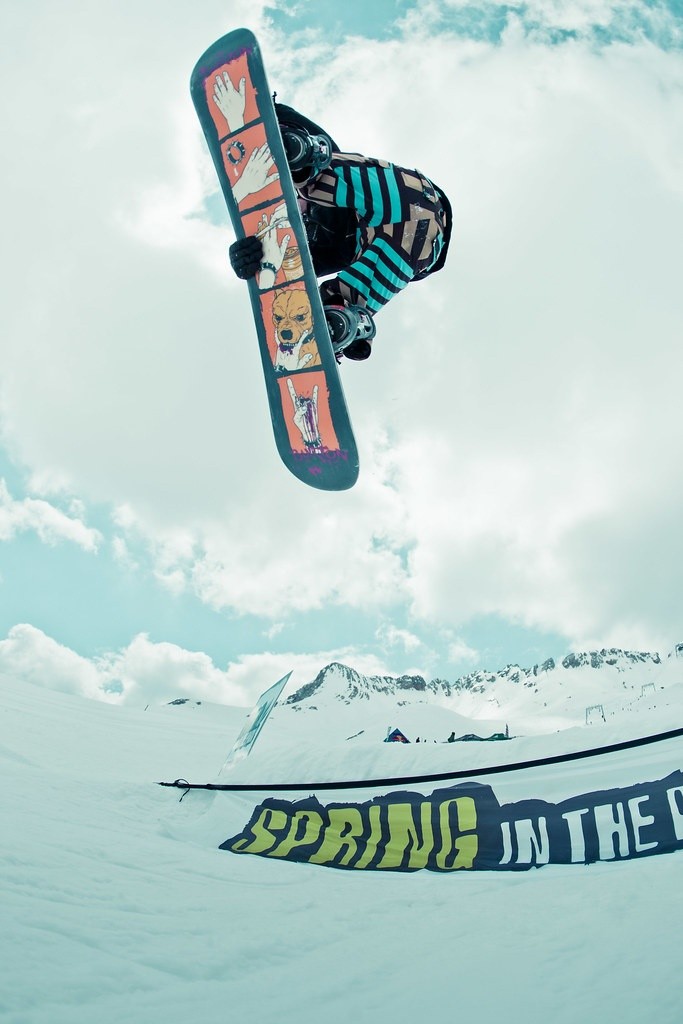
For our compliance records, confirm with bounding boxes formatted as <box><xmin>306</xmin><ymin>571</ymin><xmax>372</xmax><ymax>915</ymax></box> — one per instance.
<box><xmin>229</xmin><ymin>235</ymin><xmax>263</xmax><ymax>280</ymax></box>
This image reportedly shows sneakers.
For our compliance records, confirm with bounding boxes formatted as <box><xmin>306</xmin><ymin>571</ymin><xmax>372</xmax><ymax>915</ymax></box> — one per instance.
<box><xmin>325</xmin><ymin>309</ymin><xmax>349</xmax><ymax>354</ymax></box>
<box><xmin>280</xmin><ymin>125</ymin><xmax>309</xmax><ymax>169</ymax></box>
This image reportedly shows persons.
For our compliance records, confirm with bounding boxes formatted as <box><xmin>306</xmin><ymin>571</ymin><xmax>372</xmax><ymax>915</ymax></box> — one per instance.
<box><xmin>228</xmin><ymin>92</ymin><xmax>453</xmax><ymax>361</ymax></box>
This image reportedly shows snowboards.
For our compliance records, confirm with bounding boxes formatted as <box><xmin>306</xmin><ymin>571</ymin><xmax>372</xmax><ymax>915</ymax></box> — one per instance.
<box><xmin>188</xmin><ymin>26</ymin><xmax>361</xmax><ymax>494</ymax></box>
